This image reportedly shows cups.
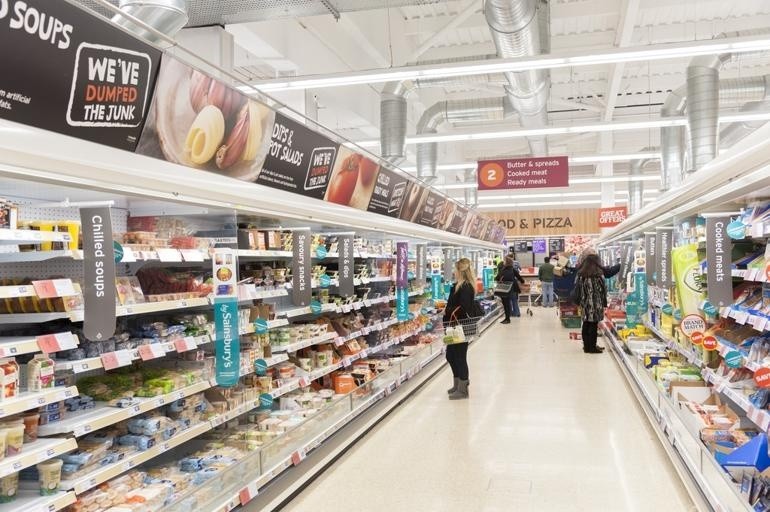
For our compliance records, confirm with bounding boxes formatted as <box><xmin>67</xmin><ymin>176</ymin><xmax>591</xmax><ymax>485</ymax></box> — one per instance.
<box><xmin>0</xmin><ymin>413</ymin><xmax>40</xmax><ymax>462</ymax></box>
<box><xmin>299</xmin><ymin>358</ymin><xmax>312</xmax><ymax>374</ymax></box>
<box><xmin>269</xmin><ymin>324</ymin><xmax>328</xmax><ymax>346</ymax></box>
<box><xmin>307</xmin><ymin>350</ymin><xmax>333</xmax><ymax>368</ymax></box>
<box><xmin>36</xmin><ymin>458</ymin><xmax>63</xmax><ymax>496</ymax></box>
<box><xmin>0</xmin><ymin>471</ymin><xmax>19</xmax><ymax>503</ymax></box>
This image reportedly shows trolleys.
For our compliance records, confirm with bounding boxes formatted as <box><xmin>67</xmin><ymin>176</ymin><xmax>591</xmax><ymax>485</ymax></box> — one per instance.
<box><xmin>518</xmin><ymin>281</ymin><xmax>558</xmax><ymax>316</ymax></box>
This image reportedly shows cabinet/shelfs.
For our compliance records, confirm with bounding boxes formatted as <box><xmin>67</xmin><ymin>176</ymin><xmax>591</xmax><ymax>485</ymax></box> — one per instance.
<box><xmin>1</xmin><ymin>116</ymin><xmax>509</xmax><ymax>512</ymax></box>
<box><xmin>592</xmin><ymin>129</ymin><xmax>770</xmax><ymax>512</ymax></box>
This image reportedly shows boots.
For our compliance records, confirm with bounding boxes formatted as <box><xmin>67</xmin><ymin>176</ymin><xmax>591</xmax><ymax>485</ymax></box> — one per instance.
<box><xmin>448</xmin><ymin>377</ymin><xmax>459</xmax><ymax>394</ymax></box>
<box><xmin>449</xmin><ymin>379</ymin><xmax>469</xmax><ymax>399</ymax></box>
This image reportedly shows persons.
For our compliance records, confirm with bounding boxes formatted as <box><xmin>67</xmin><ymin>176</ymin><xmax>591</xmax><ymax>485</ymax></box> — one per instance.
<box><xmin>579</xmin><ymin>247</ymin><xmax>621</xmax><ymax>335</ymax></box>
<box><xmin>573</xmin><ymin>254</ymin><xmax>608</xmax><ymax>354</ymax></box>
<box><xmin>492</xmin><ymin>252</ymin><xmax>526</xmax><ymax>324</ymax></box>
<box><xmin>550</xmin><ymin>254</ymin><xmax>560</xmax><ymax>268</ymax></box>
<box><xmin>435</xmin><ymin>258</ymin><xmax>476</xmax><ymax>400</ymax></box>
<box><xmin>538</xmin><ymin>257</ymin><xmax>555</xmax><ymax>308</ymax></box>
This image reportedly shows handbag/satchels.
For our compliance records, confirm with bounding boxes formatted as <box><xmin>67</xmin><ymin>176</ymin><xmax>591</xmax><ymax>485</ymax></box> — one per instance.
<box><xmin>570</xmin><ymin>276</ymin><xmax>587</xmax><ymax>306</ymax></box>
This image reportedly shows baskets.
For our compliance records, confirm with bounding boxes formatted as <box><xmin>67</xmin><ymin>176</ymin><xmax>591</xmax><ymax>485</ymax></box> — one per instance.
<box><xmin>560</xmin><ymin>315</ymin><xmax>581</xmax><ymax>328</ymax></box>
<box><xmin>433</xmin><ymin>306</ymin><xmax>483</xmax><ymax>346</ymax></box>
<box><xmin>489</xmin><ymin>277</ymin><xmax>514</xmax><ymax>293</ymax></box>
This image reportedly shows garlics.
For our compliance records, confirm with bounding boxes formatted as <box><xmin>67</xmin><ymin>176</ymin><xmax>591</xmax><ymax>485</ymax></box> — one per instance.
<box><xmin>189</xmin><ymin>74</ymin><xmax>251</xmax><ymax>168</ymax></box>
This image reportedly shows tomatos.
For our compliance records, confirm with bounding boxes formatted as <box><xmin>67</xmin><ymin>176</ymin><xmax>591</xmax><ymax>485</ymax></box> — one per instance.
<box><xmin>325</xmin><ymin>150</ymin><xmax>379</xmax><ymax>207</ymax></box>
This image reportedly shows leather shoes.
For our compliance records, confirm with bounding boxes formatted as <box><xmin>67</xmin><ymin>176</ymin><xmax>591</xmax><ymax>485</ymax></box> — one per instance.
<box><xmin>584</xmin><ymin>349</ymin><xmax>602</xmax><ymax>353</ymax></box>
<box><xmin>503</xmin><ymin>319</ymin><xmax>510</xmax><ymax>323</ymax></box>
<box><xmin>596</xmin><ymin>347</ymin><xmax>605</xmax><ymax>350</ymax></box>
<box><xmin>500</xmin><ymin>319</ymin><xmax>506</xmax><ymax>323</ymax></box>
